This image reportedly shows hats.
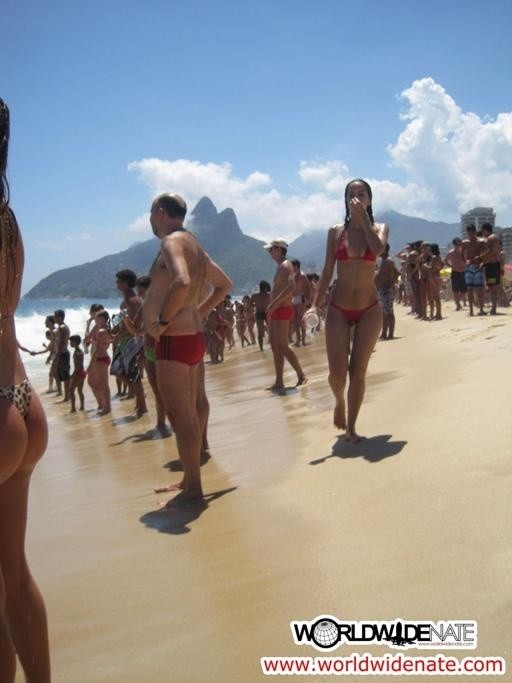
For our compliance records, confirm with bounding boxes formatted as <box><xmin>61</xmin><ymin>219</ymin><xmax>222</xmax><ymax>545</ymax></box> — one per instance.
<box><xmin>262</xmin><ymin>238</ymin><xmax>290</xmax><ymax>250</ymax></box>
<box><xmin>114</xmin><ymin>269</ymin><xmax>137</xmax><ymax>283</ymax></box>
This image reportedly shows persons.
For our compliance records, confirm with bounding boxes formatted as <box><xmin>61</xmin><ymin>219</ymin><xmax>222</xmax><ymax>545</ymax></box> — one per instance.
<box><xmin>396</xmin><ymin>237</ymin><xmax>444</xmax><ymax>323</ymax></box>
<box><xmin>52</xmin><ymin>309</ymin><xmax>70</xmax><ymax>403</ymax></box>
<box><xmin>16</xmin><ymin>335</ymin><xmax>34</xmax><ymax>358</ymax></box>
<box><xmin>111</xmin><ymin>302</ymin><xmax>128</xmax><ymax>397</ymax></box>
<box><xmin>205</xmin><ymin>239</ymin><xmax>319</xmax><ymax>391</ymax></box>
<box><xmin>32</xmin><ymin>330</ymin><xmax>53</xmax><ymax>365</ymax></box>
<box><xmin>0</xmin><ymin>99</ymin><xmax>50</xmax><ymax>683</ymax></box>
<box><xmin>69</xmin><ymin>335</ymin><xmax>87</xmax><ymax>413</ymax></box>
<box><xmin>461</xmin><ymin>223</ymin><xmax>487</xmax><ymax>317</ymax></box>
<box><xmin>110</xmin><ymin>269</ymin><xmax>148</xmax><ymax>417</ymax></box>
<box><xmin>88</xmin><ymin>310</ymin><xmax>112</xmax><ymax>414</ymax></box>
<box><xmin>499</xmin><ymin>241</ymin><xmax>510</xmax><ymax>308</ymax></box>
<box><xmin>444</xmin><ymin>236</ymin><xmax>466</xmax><ymax>312</ymax></box>
<box><xmin>122</xmin><ymin>275</ymin><xmax>168</xmax><ymax>439</ymax></box>
<box><xmin>83</xmin><ymin>303</ymin><xmax>104</xmax><ymax>410</ymax></box>
<box><xmin>479</xmin><ymin>223</ymin><xmax>500</xmax><ymax>317</ymax></box>
<box><xmin>142</xmin><ymin>192</ymin><xmax>232</xmax><ymax>507</ymax></box>
<box><xmin>316</xmin><ymin>179</ymin><xmax>389</xmax><ymax>445</ymax></box>
<box><xmin>375</xmin><ymin>244</ymin><xmax>399</xmax><ymax>341</ymax></box>
<box><xmin>45</xmin><ymin>316</ymin><xmax>59</xmax><ymax>394</ymax></box>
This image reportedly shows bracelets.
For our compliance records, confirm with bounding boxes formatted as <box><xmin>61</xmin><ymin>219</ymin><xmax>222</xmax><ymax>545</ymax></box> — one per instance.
<box><xmin>155</xmin><ymin>311</ymin><xmax>170</xmax><ymax>327</ymax></box>
